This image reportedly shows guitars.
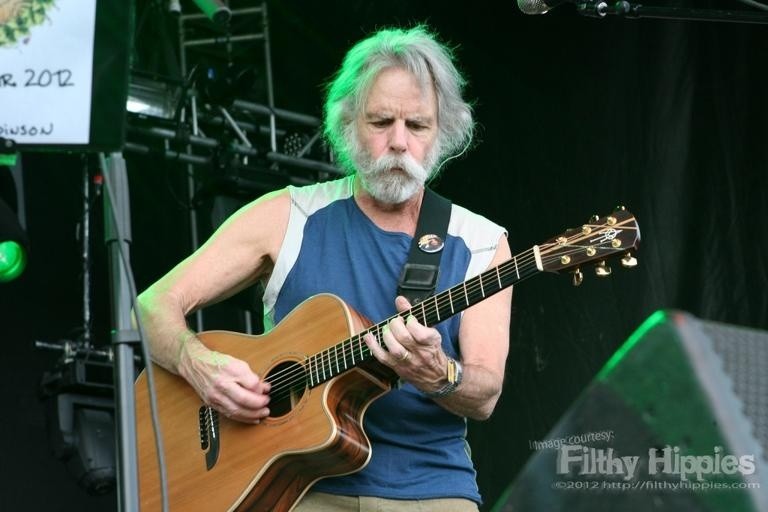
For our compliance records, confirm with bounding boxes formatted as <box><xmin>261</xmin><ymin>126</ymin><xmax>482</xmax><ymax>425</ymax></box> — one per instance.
<box><xmin>133</xmin><ymin>205</ymin><xmax>642</xmax><ymax>512</ymax></box>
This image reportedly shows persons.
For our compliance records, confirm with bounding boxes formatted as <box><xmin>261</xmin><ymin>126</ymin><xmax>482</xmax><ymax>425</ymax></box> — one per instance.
<box><xmin>130</xmin><ymin>24</ymin><xmax>515</xmax><ymax>512</ymax></box>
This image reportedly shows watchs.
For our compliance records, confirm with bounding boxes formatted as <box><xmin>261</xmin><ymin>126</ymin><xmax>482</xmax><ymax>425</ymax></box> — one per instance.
<box><xmin>420</xmin><ymin>356</ymin><xmax>464</xmax><ymax>398</ymax></box>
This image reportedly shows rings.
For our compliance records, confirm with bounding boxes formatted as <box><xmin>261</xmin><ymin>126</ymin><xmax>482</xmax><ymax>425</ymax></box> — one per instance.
<box><xmin>397</xmin><ymin>349</ymin><xmax>409</xmax><ymax>363</ymax></box>
